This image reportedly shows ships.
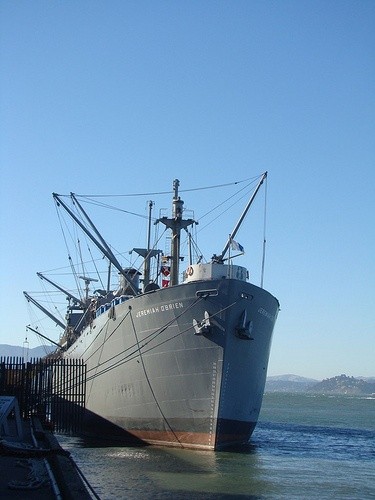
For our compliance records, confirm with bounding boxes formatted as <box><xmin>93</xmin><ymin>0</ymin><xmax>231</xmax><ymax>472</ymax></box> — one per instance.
<box><xmin>26</xmin><ymin>170</ymin><xmax>281</xmax><ymax>453</ymax></box>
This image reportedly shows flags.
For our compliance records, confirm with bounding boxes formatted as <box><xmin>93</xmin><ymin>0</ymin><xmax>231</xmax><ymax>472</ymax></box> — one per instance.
<box><xmin>161</xmin><ymin>266</ymin><xmax>170</xmax><ymax>287</ymax></box>
<box><xmin>231</xmin><ymin>239</ymin><xmax>244</xmax><ymax>252</ymax></box>
